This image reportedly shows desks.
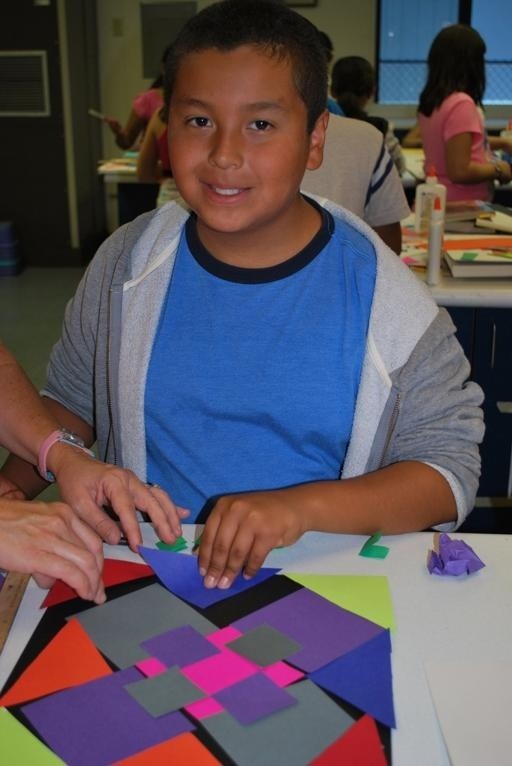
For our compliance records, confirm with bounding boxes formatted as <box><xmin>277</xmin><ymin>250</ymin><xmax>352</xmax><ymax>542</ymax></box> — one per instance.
<box><xmin>98</xmin><ymin>155</ymin><xmax>163</xmax><ymax>237</ymax></box>
<box><xmin>409</xmin><ymin>166</ymin><xmax>512</xmax><ymax>506</ymax></box>
<box><xmin>1</xmin><ymin>523</ymin><xmax>511</xmax><ymax>766</ymax></box>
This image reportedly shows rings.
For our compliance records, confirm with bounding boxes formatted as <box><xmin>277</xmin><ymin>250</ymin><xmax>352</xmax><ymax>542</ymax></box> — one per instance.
<box><xmin>144</xmin><ymin>483</ymin><xmax>162</xmax><ymax>488</ymax></box>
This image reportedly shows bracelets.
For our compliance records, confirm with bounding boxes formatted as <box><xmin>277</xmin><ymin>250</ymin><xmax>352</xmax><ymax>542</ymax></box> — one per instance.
<box><xmin>37</xmin><ymin>426</ymin><xmax>98</xmax><ymax>482</ymax></box>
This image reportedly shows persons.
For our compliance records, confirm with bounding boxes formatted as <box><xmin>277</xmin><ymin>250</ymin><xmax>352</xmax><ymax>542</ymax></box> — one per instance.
<box><xmin>0</xmin><ymin>2</ymin><xmax>487</xmax><ymax>591</ymax></box>
<box><xmin>299</xmin><ymin>29</ymin><xmax>412</xmax><ymax>256</ymax></box>
<box><xmin>0</xmin><ymin>344</ymin><xmax>191</xmax><ymax>606</ymax></box>
<box><xmin>418</xmin><ymin>23</ymin><xmax>512</xmax><ymax>202</ymax></box>
<box><xmin>108</xmin><ymin>73</ymin><xmax>163</xmax><ymax>150</ymax></box>
<box><xmin>137</xmin><ymin>101</ymin><xmax>182</xmax><ymax>207</ymax></box>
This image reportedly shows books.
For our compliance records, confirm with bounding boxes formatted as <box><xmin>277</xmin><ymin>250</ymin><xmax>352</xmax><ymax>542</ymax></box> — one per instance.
<box><xmin>398</xmin><ymin>196</ymin><xmax>511</xmax><ymax>279</ymax></box>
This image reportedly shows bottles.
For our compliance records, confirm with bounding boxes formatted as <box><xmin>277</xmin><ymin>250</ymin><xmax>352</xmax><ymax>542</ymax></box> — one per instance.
<box><xmin>425</xmin><ymin>196</ymin><xmax>444</xmax><ymax>287</ymax></box>
<box><xmin>414</xmin><ymin>165</ymin><xmax>447</xmax><ymax>237</ymax></box>
